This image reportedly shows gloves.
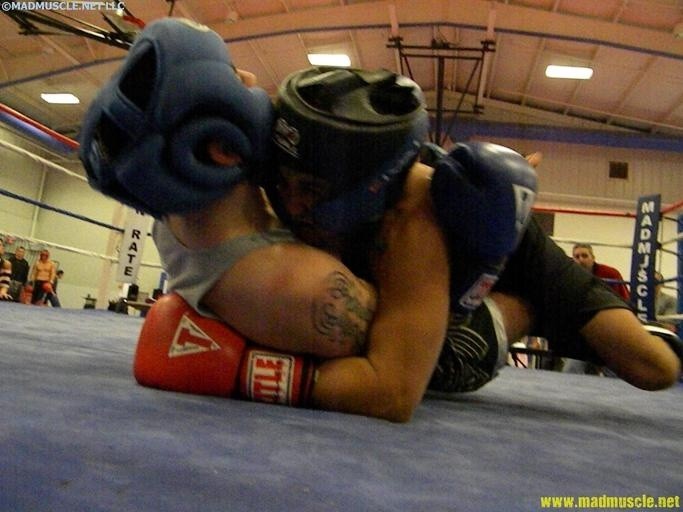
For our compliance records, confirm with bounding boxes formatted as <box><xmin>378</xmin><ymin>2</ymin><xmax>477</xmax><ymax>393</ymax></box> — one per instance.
<box><xmin>427</xmin><ymin>140</ymin><xmax>538</xmax><ymax>315</ymax></box>
<box><xmin>43</xmin><ymin>280</ymin><xmax>54</xmax><ymax>292</ymax></box>
<box><xmin>25</xmin><ymin>281</ymin><xmax>33</xmax><ymax>292</ymax></box>
<box><xmin>133</xmin><ymin>292</ymin><xmax>321</xmax><ymax>408</ymax></box>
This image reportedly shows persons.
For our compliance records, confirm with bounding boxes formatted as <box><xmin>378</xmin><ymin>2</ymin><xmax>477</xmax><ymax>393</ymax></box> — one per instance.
<box><xmin>563</xmin><ymin>244</ymin><xmax>632</xmax><ymax>376</ymax></box>
<box><xmin>0</xmin><ymin>240</ymin><xmax>67</xmax><ymax>308</ymax></box>
<box><xmin>133</xmin><ymin>68</ymin><xmax>683</xmax><ymax>422</ymax></box>
<box><xmin>652</xmin><ymin>270</ymin><xmax>678</xmax><ymax>332</ymax></box>
<box><xmin>77</xmin><ymin>20</ymin><xmax>544</xmax><ymax>394</ymax></box>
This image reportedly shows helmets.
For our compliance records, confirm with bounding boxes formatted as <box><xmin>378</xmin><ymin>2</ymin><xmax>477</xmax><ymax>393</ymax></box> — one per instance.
<box><xmin>76</xmin><ymin>14</ymin><xmax>278</xmax><ymax>217</ymax></box>
<box><xmin>261</xmin><ymin>65</ymin><xmax>429</xmax><ymax>240</ymax></box>
